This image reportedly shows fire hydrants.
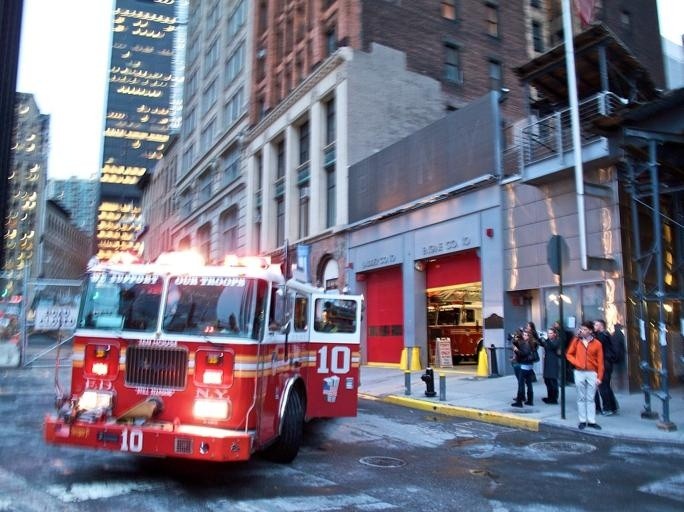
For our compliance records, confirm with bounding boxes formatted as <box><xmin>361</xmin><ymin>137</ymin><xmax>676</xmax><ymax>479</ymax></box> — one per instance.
<box><xmin>421</xmin><ymin>367</ymin><xmax>436</xmax><ymax>396</ymax></box>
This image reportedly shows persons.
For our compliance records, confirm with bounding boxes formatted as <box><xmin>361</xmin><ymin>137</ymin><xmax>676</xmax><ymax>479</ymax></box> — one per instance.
<box><xmin>509</xmin><ymin>319</ymin><xmax>619</xmax><ymax>430</ymax></box>
<box><xmin>611</xmin><ymin>323</ymin><xmax>626</xmax><ymax>392</ymax></box>
<box><xmin>4</xmin><ymin>318</ymin><xmax>18</xmax><ymax>339</ymax></box>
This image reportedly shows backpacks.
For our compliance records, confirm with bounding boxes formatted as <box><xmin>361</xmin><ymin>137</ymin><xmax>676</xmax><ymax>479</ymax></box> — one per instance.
<box><xmin>603</xmin><ymin>331</ymin><xmax>625</xmax><ymax>363</ymax></box>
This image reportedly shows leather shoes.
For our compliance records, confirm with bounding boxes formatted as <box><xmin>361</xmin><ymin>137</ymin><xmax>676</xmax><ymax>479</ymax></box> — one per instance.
<box><xmin>513</xmin><ymin>398</ymin><xmax>526</xmax><ymax>401</ymax></box>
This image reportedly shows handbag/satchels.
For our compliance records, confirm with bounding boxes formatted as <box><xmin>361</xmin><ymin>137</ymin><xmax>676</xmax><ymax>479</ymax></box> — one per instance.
<box><xmin>521</xmin><ymin>349</ymin><xmax>539</xmax><ymax>362</ymax></box>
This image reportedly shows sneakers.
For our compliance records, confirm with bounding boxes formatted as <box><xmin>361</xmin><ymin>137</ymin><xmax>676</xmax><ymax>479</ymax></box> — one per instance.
<box><xmin>588</xmin><ymin>423</ymin><xmax>601</xmax><ymax>429</ymax></box>
<box><xmin>596</xmin><ymin>408</ymin><xmax>617</xmax><ymax>415</ymax></box>
<box><xmin>579</xmin><ymin>423</ymin><xmax>586</xmax><ymax>429</ymax></box>
<box><xmin>542</xmin><ymin>397</ymin><xmax>557</xmax><ymax>404</ymax></box>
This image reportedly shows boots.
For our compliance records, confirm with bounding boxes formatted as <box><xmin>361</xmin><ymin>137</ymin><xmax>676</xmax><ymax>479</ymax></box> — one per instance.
<box><xmin>525</xmin><ymin>392</ymin><xmax>533</xmax><ymax>405</ymax></box>
<box><xmin>512</xmin><ymin>394</ymin><xmax>522</xmax><ymax>406</ymax></box>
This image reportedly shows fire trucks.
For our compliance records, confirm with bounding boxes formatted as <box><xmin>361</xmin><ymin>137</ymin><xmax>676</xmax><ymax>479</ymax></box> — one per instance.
<box><xmin>427</xmin><ymin>299</ymin><xmax>484</xmax><ymax>363</ymax></box>
<box><xmin>41</xmin><ymin>248</ymin><xmax>364</xmax><ymax>463</ymax></box>
